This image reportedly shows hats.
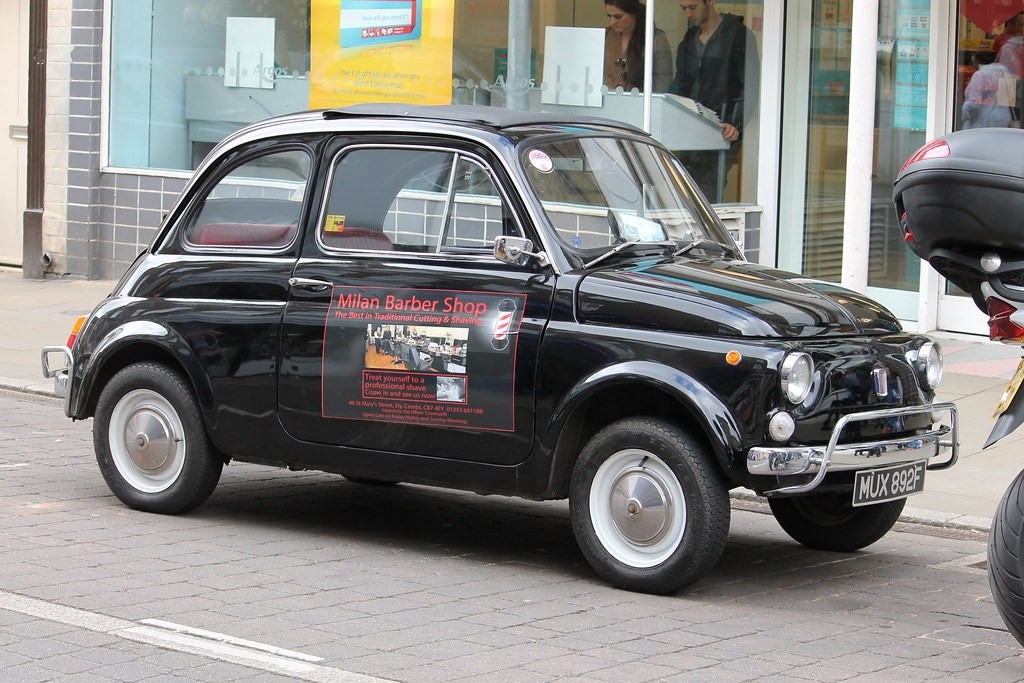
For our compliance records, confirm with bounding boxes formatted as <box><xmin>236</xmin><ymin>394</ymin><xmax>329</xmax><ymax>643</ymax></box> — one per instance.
<box><xmin>976</xmin><ymin>52</ymin><xmax>996</xmax><ymax>65</ymax></box>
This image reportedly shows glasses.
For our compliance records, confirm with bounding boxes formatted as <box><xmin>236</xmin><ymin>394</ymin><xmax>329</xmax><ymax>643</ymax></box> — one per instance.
<box><xmin>616</xmin><ymin>57</ymin><xmax>629</xmax><ymax>82</ymax></box>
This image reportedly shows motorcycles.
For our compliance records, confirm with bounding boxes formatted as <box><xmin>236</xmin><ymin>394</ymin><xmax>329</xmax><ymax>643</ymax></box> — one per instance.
<box><xmin>891</xmin><ymin>126</ymin><xmax>1024</xmax><ymax>650</ymax></box>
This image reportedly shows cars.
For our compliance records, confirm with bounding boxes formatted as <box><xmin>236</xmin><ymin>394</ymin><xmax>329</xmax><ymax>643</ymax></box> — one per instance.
<box><xmin>41</xmin><ymin>102</ymin><xmax>958</xmax><ymax>596</ymax></box>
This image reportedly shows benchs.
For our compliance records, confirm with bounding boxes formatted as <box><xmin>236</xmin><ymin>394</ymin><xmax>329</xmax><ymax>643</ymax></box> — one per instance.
<box><xmin>191</xmin><ymin>221</ymin><xmax>393</xmax><ymax>245</ymax></box>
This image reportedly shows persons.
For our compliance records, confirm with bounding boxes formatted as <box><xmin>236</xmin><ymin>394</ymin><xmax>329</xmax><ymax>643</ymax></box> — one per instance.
<box><xmin>671</xmin><ymin>0</ymin><xmax>760</xmax><ymax>204</ymax></box>
<box><xmin>374</xmin><ymin>326</ymin><xmax>391</xmax><ymax>355</ymax></box>
<box><xmin>604</xmin><ymin>0</ymin><xmax>672</xmax><ymax>94</ymax></box>
<box><xmin>962</xmin><ymin>11</ymin><xmax>1024</xmax><ymax>130</ymax></box>
<box><xmin>412</xmin><ymin>329</ymin><xmax>426</xmax><ymax>337</ymax></box>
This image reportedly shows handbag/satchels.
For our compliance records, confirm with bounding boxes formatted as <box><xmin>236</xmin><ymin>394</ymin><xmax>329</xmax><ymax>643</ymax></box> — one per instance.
<box><xmin>1011</xmin><ymin>120</ymin><xmax>1021</xmax><ymax>128</ymax></box>
